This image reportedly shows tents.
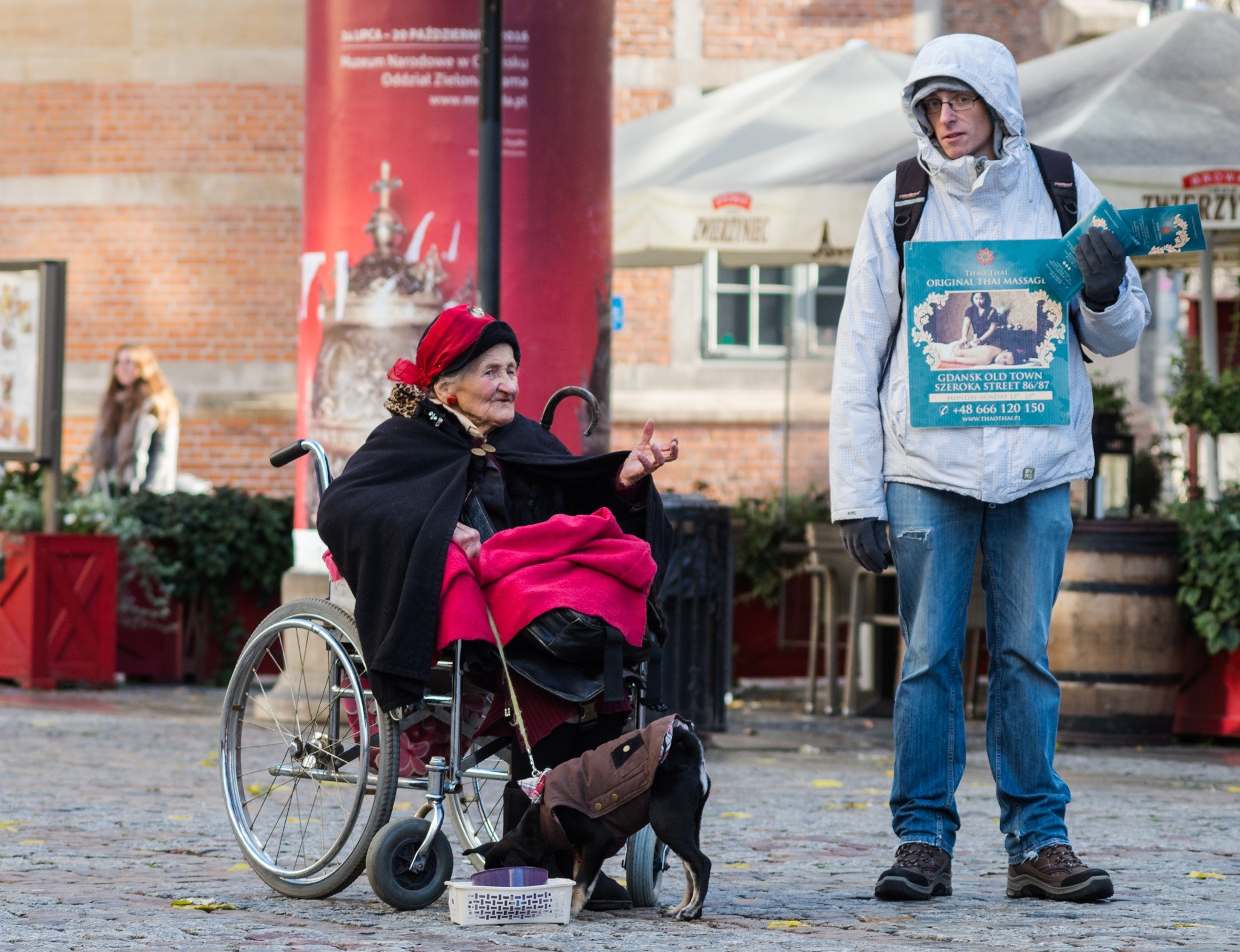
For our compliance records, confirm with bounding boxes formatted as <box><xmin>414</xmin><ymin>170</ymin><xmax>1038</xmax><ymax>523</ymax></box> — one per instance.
<box><xmin>612</xmin><ymin>6</ymin><xmax>1239</xmax><ymax>517</ymax></box>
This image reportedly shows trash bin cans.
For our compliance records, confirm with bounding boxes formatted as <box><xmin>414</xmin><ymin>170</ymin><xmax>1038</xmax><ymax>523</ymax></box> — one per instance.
<box><xmin>646</xmin><ymin>489</ymin><xmax>732</xmax><ymax>733</ymax></box>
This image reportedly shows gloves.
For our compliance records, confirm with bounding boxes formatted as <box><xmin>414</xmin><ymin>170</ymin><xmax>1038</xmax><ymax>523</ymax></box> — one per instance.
<box><xmin>1076</xmin><ymin>226</ymin><xmax>1126</xmax><ymax>302</ymax></box>
<box><xmin>839</xmin><ymin>518</ymin><xmax>890</xmax><ymax>574</ymax></box>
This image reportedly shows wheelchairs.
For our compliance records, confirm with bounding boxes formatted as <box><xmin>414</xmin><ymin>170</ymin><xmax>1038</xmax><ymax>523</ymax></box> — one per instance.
<box><xmin>216</xmin><ymin>439</ymin><xmax>673</xmax><ymax>913</ymax></box>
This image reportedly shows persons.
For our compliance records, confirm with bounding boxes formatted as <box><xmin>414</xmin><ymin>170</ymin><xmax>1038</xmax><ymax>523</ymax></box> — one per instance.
<box><xmin>88</xmin><ymin>342</ymin><xmax>179</xmax><ymax>497</ymax></box>
<box><xmin>830</xmin><ymin>32</ymin><xmax>1150</xmax><ymax>901</ymax></box>
<box><xmin>316</xmin><ymin>303</ymin><xmax>680</xmax><ymax>912</ymax></box>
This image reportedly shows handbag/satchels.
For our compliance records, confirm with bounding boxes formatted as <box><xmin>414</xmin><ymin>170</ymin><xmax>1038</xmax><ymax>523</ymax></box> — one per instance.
<box><xmin>525</xmin><ymin>605</ymin><xmax>654</xmax><ymax>668</ymax></box>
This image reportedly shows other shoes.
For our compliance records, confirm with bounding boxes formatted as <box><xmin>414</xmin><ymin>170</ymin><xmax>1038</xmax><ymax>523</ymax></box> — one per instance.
<box><xmin>585</xmin><ymin>871</ymin><xmax>631</xmax><ymax>912</ymax></box>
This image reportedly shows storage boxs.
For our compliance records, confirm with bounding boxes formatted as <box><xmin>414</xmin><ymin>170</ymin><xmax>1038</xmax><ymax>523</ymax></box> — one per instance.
<box><xmin>444</xmin><ymin>878</ymin><xmax>576</xmax><ymax>926</ymax></box>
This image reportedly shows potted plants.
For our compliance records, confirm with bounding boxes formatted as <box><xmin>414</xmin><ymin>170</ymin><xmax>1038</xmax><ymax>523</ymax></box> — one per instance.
<box><xmin>0</xmin><ymin>458</ymin><xmax>119</xmax><ymax>690</ymax></box>
<box><xmin>1046</xmin><ymin>382</ymin><xmax>1188</xmax><ymax>744</ymax></box>
<box><xmin>731</xmin><ymin>484</ymin><xmax>846</xmax><ymax>677</ymax></box>
<box><xmin>1167</xmin><ymin>482</ymin><xmax>1240</xmax><ymax>738</ymax></box>
<box><xmin>114</xmin><ymin>485</ymin><xmax>294</xmax><ymax>685</ymax></box>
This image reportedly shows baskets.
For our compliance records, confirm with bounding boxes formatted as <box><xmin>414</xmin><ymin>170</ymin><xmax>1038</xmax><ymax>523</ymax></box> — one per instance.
<box><xmin>443</xmin><ymin>877</ymin><xmax>576</xmax><ymax>926</ymax></box>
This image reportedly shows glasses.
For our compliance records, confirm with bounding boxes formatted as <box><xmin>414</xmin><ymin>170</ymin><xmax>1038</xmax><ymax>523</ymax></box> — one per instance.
<box><xmin>916</xmin><ymin>95</ymin><xmax>981</xmax><ymax>114</ymax></box>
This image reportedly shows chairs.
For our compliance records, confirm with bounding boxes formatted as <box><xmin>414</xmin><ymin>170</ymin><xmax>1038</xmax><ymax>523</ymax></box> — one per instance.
<box><xmin>803</xmin><ymin>524</ymin><xmax>871</xmax><ymax>715</ymax></box>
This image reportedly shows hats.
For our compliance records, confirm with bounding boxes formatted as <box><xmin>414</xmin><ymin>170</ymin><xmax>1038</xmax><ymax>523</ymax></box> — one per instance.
<box><xmin>416</xmin><ymin>304</ymin><xmax>521</xmax><ymax>393</ymax></box>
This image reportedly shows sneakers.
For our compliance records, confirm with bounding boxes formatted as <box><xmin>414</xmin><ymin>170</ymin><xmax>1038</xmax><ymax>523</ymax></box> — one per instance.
<box><xmin>874</xmin><ymin>843</ymin><xmax>954</xmax><ymax>899</ymax></box>
<box><xmin>1006</xmin><ymin>844</ymin><xmax>1113</xmax><ymax>901</ymax></box>
